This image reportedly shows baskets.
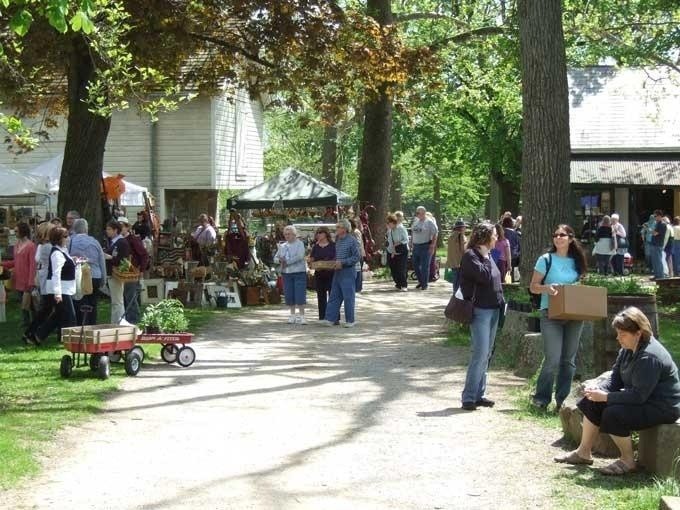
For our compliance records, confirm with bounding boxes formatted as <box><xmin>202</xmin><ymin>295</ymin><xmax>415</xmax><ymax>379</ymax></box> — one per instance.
<box><xmin>112</xmin><ymin>265</ymin><xmax>141</xmax><ymax>283</ymax></box>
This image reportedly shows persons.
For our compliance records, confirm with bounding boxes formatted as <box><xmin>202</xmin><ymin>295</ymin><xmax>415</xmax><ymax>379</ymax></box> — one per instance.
<box><xmin>459</xmin><ymin>223</ymin><xmax>508</xmax><ymax>409</ymax></box>
<box><xmin>273</xmin><ymin>224</ymin><xmax>308</xmax><ymax>325</ymax></box>
<box><xmin>530</xmin><ymin>224</ymin><xmax>587</xmax><ymax>410</ymax></box>
<box><xmin>386</xmin><ymin>206</ymin><xmax>680</xmax><ymax>291</ymax></box>
<box><xmin>307</xmin><ymin>227</ymin><xmax>342</xmax><ymax>325</ymax></box>
<box><xmin>350</xmin><ymin>220</ymin><xmax>368</xmax><ymax>298</ymax></box>
<box><xmin>554</xmin><ymin>307</ymin><xmax>680</xmax><ymax>474</ymax></box>
<box><xmin>315</xmin><ymin>219</ymin><xmax>361</xmax><ymax>328</ymax></box>
<box><xmin>0</xmin><ymin>204</ymin><xmax>221</xmax><ymax>347</ymax></box>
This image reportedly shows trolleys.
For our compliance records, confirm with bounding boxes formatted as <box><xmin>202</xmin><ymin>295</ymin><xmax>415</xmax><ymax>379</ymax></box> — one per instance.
<box><xmin>52</xmin><ymin>315</ymin><xmax>199</xmax><ymax>381</ymax></box>
<box><xmin>595</xmin><ymin>253</ymin><xmax>635</xmax><ymax>276</ymax></box>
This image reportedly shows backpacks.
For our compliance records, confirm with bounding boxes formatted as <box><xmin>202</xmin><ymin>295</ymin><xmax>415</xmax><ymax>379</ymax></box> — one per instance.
<box><xmin>395</xmin><ymin>244</ymin><xmax>407</xmax><ymax>253</ymax></box>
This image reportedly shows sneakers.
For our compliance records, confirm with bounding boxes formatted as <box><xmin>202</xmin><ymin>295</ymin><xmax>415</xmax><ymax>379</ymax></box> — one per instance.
<box><xmin>395</xmin><ymin>283</ymin><xmax>429</xmax><ymax>292</ymax></box>
<box><xmin>287</xmin><ymin>315</ymin><xmax>357</xmax><ymax>328</ymax></box>
<box><xmin>463</xmin><ymin>398</ymin><xmax>565</xmax><ymax>415</ymax></box>
<box><xmin>17</xmin><ymin>320</ymin><xmax>61</xmax><ymax>345</ymax></box>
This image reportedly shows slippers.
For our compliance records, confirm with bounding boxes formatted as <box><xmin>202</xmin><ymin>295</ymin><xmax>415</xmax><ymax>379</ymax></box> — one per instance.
<box><xmin>554</xmin><ymin>450</ymin><xmax>593</xmax><ymax>464</ymax></box>
<box><xmin>598</xmin><ymin>460</ymin><xmax>637</xmax><ymax>475</ymax></box>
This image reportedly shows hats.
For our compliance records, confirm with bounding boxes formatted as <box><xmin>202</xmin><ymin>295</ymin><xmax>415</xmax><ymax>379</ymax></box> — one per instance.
<box><xmin>453</xmin><ymin>222</ymin><xmax>469</xmax><ymax>228</ymax></box>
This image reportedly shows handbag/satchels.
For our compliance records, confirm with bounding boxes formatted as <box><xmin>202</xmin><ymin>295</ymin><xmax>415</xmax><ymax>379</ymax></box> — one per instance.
<box><xmin>21</xmin><ymin>263</ymin><xmax>94</xmax><ymax>312</ymax></box>
<box><xmin>445</xmin><ymin>296</ymin><xmax>474</xmax><ymax>323</ymax></box>
<box><xmin>618</xmin><ymin>237</ymin><xmax>629</xmax><ymax>248</ymax></box>
<box><xmin>578</xmin><ymin>370</ymin><xmax>614</xmax><ymax>392</ymax></box>
